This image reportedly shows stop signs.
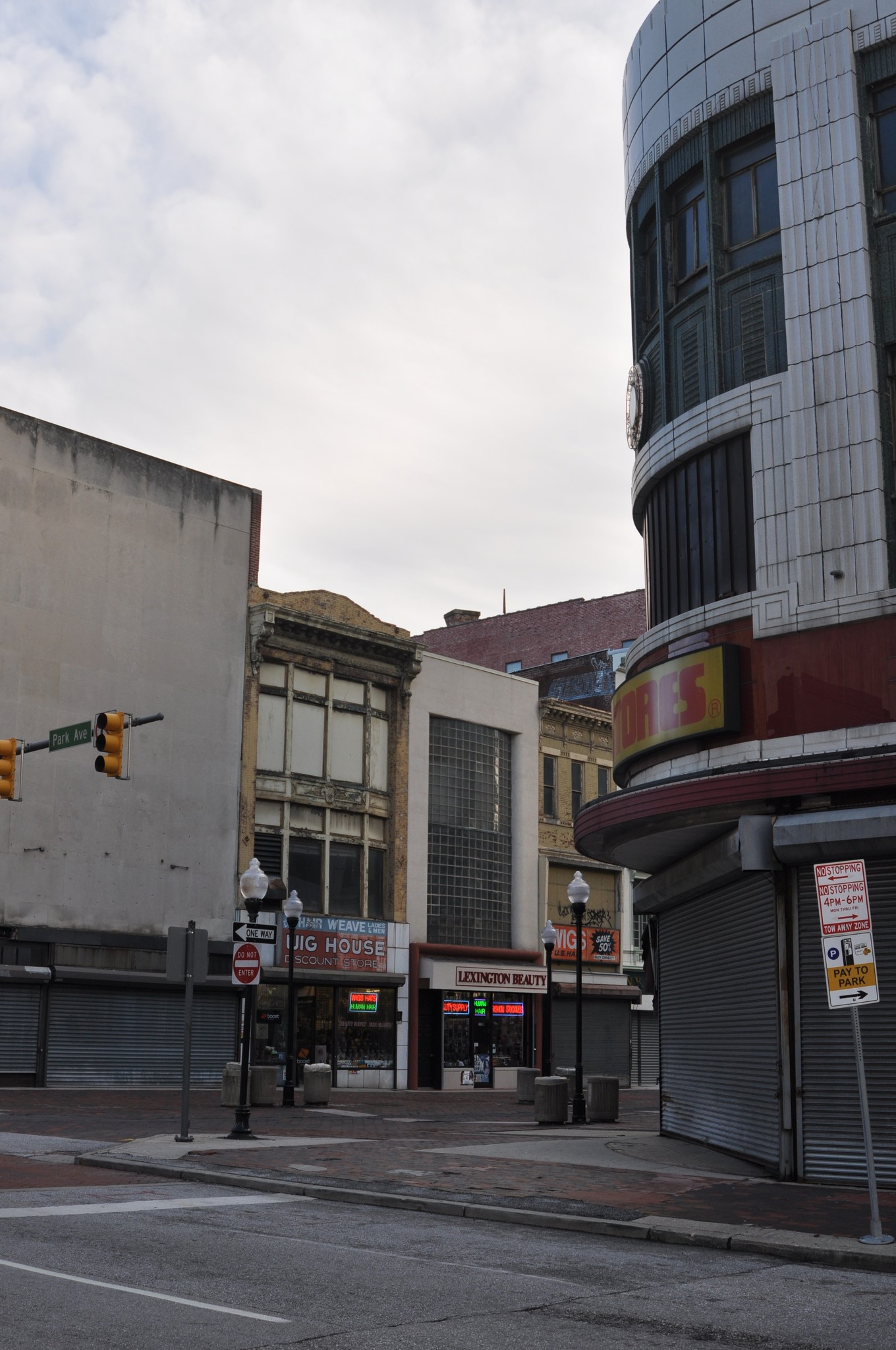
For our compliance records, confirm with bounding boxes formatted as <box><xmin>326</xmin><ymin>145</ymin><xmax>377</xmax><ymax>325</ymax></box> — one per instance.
<box><xmin>232</xmin><ymin>943</ymin><xmax>262</xmax><ymax>985</ymax></box>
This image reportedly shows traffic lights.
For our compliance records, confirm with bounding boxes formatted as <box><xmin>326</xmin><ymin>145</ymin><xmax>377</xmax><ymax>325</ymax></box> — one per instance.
<box><xmin>0</xmin><ymin>737</ymin><xmax>17</xmax><ymax>800</ymax></box>
<box><xmin>95</xmin><ymin>711</ymin><xmax>125</xmax><ymax>778</ymax></box>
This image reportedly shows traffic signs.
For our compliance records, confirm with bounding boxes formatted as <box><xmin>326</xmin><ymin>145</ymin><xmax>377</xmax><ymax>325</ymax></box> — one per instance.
<box><xmin>821</xmin><ymin>930</ymin><xmax>880</xmax><ymax>1010</ymax></box>
<box><xmin>232</xmin><ymin>921</ymin><xmax>278</xmax><ymax>946</ymax></box>
<box><xmin>813</xmin><ymin>858</ymin><xmax>873</xmax><ymax>937</ymax></box>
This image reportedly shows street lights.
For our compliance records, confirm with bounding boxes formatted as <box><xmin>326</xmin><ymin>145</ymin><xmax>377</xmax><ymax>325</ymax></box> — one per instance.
<box><xmin>540</xmin><ymin>918</ymin><xmax>557</xmax><ymax>1076</ymax></box>
<box><xmin>565</xmin><ymin>870</ymin><xmax>592</xmax><ymax>1125</ymax></box>
<box><xmin>284</xmin><ymin>889</ymin><xmax>304</xmax><ymax>1108</ymax></box>
<box><xmin>227</xmin><ymin>857</ymin><xmax>269</xmax><ymax>1140</ymax></box>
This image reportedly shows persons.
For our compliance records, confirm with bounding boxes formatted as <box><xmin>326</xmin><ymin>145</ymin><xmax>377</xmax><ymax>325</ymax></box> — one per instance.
<box><xmin>469</xmin><ymin>1071</ymin><xmax>473</xmax><ymax>1084</ymax></box>
<box><xmin>345</xmin><ymin>1028</ymin><xmax>354</xmax><ymax>1045</ymax></box>
<box><xmin>474</xmin><ymin>1062</ymin><xmax>480</xmax><ymax>1070</ymax></box>
<box><xmin>483</xmin><ymin>1059</ymin><xmax>489</xmax><ymax>1074</ymax></box>
<box><xmin>461</xmin><ymin>1071</ymin><xmax>465</xmax><ymax>1084</ymax></box>
<box><xmin>317</xmin><ymin>1029</ymin><xmax>331</xmax><ymax>1054</ymax></box>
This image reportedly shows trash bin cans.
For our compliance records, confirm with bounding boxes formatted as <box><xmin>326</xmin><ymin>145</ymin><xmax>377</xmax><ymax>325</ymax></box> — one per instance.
<box><xmin>303</xmin><ymin>1064</ymin><xmax>333</xmax><ymax>1108</ymax></box>
<box><xmin>517</xmin><ymin>1068</ymin><xmax>541</xmax><ymax>1104</ymax></box>
<box><xmin>556</xmin><ymin>1067</ymin><xmax>575</xmax><ymax>1104</ymax></box>
<box><xmin>587</xmin><ymin>1076</ymin><xmax>619</xmax><ymax>1123</ymax></box>
<box><xmin>249</xmin><ymin>1066</ymin><xmax>278</xmax><ymax>1107</ymax></box>
<box><xmin>220</xmin><ymin>1062</ymin><xmax>251</xmax><ymax>1107</ymax></box>
<box><xmin>535</xmin><ymin>1076</ymin><xmax>568</xmax><ymax>1127</ymax></box>
